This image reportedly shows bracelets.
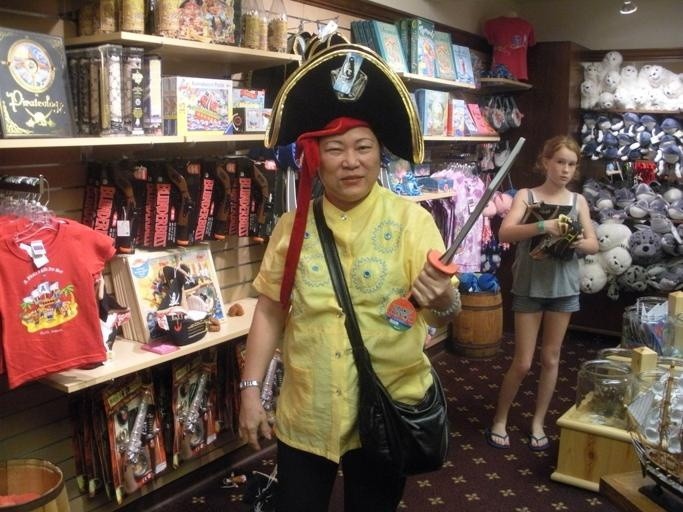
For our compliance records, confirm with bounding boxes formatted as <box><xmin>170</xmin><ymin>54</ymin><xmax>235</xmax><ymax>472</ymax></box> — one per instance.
<box><xmin>432</xmin><ymin>286</ymin><xmax>462</xmax><ymax>318</ymax></box>
<box><xmin>238</xmin><ymin>379</ymin><xmax>263</xmax><ymax>390</ymax></box>
<box><xmin>537</xmin><ymin>221</ymin><xmax>546</xmax><ymax>233</ymax></box>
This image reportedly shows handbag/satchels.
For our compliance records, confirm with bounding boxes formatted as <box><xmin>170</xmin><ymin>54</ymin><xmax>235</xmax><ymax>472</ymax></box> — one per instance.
<box><xmin>357</xmin><ymin>368</ymin><xmax>450</xmax><ymax>480</ymax></box>
<box><xmin>495</xmin><ymin>141</ymin><xmax>511</xmax><ymax>167</ymax></box>
<box><xmin>547</xmin><ymin>221</ymin><xmax>583</xmax><ymax>261</ymax></box>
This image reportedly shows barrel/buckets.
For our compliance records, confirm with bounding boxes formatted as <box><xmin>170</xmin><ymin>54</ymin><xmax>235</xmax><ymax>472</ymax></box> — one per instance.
<box><xmin>0</xmin><ymin>456</ymin><xmax>70</xmax><ymax>511</ymax></box>
<box><xmin>448</xmin><ymin>293</ymin><xmax>505</xmax><ymax>358</ymax></box>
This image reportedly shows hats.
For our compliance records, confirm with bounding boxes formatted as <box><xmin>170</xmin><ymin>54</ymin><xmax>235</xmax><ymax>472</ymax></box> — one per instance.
<box><xmin>263</xmin><ymin>32</ymin><xmax>425</xmax><ymax>165</ymax></box>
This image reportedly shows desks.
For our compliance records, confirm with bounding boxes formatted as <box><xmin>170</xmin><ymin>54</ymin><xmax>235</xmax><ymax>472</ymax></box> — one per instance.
<box><xmin>596</xmin><ymin>473</ymin><xmax>681</xmax><ymax>512</ymax></box>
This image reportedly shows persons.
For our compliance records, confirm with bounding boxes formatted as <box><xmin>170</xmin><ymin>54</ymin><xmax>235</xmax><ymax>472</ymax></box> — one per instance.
<box><xmin>235</xmin><ymin>105</ymin><xmax>465</xmax><ymax>512</ymax></box>
<box><xmin>483</xmin><ymin>11</ymin><xmax>536</xmax><ymax>81</ymax></box>
<box><xmin>484</xmin><ymin>131</ymin><xmax>602</xmax><ymax>451</ymax></box>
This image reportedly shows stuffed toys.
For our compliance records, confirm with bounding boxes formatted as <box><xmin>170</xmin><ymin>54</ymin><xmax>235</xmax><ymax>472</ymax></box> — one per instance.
<box><xmin>572</xmin><ymin>48</ymin><xmax>683</xmax><ymax>302</ymax></box>
<box><xmin>460</xmin><ymin>181</ymin><xmax>523</xmax><ymax>297</ymax></box>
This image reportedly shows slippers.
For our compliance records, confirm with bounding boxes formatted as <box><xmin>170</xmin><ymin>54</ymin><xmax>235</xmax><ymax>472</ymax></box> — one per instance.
<box><xmin>486</xmin><ymin>427</ymin><xmax>510</xmax><ymax>449</ymax></box>
<box><xmin>527</xmin><ymin>432</ymin><xmax>549</xmax><ymax>452</ymax></box>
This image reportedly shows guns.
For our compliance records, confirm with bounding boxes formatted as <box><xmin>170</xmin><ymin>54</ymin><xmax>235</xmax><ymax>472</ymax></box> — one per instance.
<box><xmin>179</xmin><ymin>373</ymin><xmax>209</xmax><ymax>461</ymax></box>
<box><xmin>120</xmin><ymin>390</ymin><xmax>148</xmax><ymax>494</ymax></box>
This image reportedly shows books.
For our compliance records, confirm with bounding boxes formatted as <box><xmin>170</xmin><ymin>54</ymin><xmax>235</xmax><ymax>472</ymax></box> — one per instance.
<box><xmin>345</xmin><ymin>13</ymin><xmax>496</xmax><ymax>196</ymax></box>
<box><xmin>0</xmin><ymin>2</ymin><xmax>287</xmax><ymax>147</ymax></box>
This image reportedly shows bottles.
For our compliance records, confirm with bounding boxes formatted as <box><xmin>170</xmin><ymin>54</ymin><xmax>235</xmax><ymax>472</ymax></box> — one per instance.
<box><xmin>573</xmin><ymin>345</ymin><xmax>637</xmax><ymax>426</ymax></box>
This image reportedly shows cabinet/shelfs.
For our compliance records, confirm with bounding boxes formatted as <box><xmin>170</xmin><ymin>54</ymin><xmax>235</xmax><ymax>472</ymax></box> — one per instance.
<box><xmin>2</xmin><ymin>2</ymin><xmax>534</xmax><ymax>510</ymax></box>
<box><xmin>536</xmin><ymin>41</ymin><xmax>683</xmax><ymax>335</ymax></box>
<box><xmin>548</xmin><ymin>371</ymin><xmax>668</xmax><ymax>492</ymax></box>
